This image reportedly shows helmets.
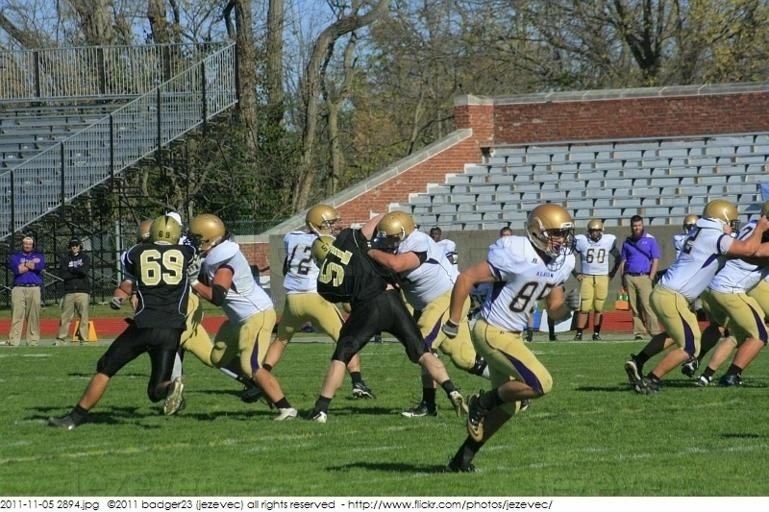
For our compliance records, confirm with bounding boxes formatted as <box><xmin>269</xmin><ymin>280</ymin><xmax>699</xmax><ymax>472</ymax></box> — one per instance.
<box><xmin>526</xmin><ymin>203</ymin><xmax>578</xmax><ymax>260</ymax></box>
<box><xmin>703</xmin><ymin>200</ymin><xmax>740</xmax><ymax>235</ymax></box>
<box><xmin>305</xmin><ymin>204</ymin><xmax>338</xmax><ymax>235</ymax></box>
<box><xmin>586</xmin><ymin>219</ymin><xmax>604</xmax><ymax>231</ymax></box>
<box><xmin>187</xmin><ymin>213</ymin><xmax>227</xmax><ymax>252</ymax></box>
<box><xmin>149</xmin><ymin>215</ymin><xmax>181</xmax><ymax>245</ymax></box>
<box><xmin>311</xmin><ymin>236</ymin><xmax>334</xmax><ymax>268</ymax></box>
<box><xmin>760</xmin><ymin>200</ymin><xmax>769</xmax><ymax>218</ymax></box>
<box><xmin>135</xmin><ymin>219</ymin><xmax>154</xmax><ymax>243</ymax></box>
<box><xmin>377</xmin><ymin>212</ymin><xmax>415</xmax><ymax>252</ymax></box>
<box><xmin>682</xmin><ymin>215</ymin><xmax>699</xmax><ymax>233</ymax></box>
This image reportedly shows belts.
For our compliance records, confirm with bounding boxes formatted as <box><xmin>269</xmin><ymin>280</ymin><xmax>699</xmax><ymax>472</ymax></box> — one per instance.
<box><xmin>627</xmin><ymin>273</ymin><xmax>649</xmax><ymax>277</ymax></box>
<box><xmin>14</xmin><ymin>284</ymin><xmax>40</xmax><ymax>289</ymax></box>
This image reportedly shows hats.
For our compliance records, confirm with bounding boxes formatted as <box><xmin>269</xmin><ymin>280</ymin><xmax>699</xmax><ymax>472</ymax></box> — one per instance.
<box><xmin>69</xmin><ymin>237</ymin><xmax>80</xmax><ymax>246</ymax></box>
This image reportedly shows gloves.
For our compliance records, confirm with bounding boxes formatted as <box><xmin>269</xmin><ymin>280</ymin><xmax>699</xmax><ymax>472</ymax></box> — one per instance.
<box><xmin>565</xmin><ymin>288</ymin><xmax>582</xmax><ymax>312</ymax></box>
<box><xmin>442</xmin><ymin>319</ymin><xmax>459</xmax><ymax>340</ymax></box>
<box><xmin>109</xmin><ymin>296</ymin><xmax>123</xmax><ymax>310</ymax></box>
<box><xmin>186</xmin><ymin>262</ymin><xmax>201</xmax><ymax>286</ymax></box>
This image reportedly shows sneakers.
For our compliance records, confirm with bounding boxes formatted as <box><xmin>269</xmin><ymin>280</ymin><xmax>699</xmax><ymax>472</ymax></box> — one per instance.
<box><xmin>352</xmin><ymin>381</ymin><xmax>375</xmax><ymax>399</ymax></box>
<box><xmin>548</xmin><ymin>335</ymin><xmax>559</xmax><ymax>341</ymax></box>
<box><xmin>302</xmin><ymin>410</ymin><xmax>328</xmax><ymax>423</ymax></box>
<box><xmin>623</xmin><ymin>357</ymin><xmax>643</xmax><ymax>383</ymax></box>
<box><xmin>681</xmin><ymin>357</ymin><xmax>699</xmax><ymax>378</ymax></box>
<box><xmin>718</xmin><ymin>374</ymin><xmax>742</xmax><ymax>386</ymax></box>
<box><xmin>52</xmin><ymin>339</ymin><xmax>64</xmax><ymax>346</ymax></box>
<box><xmin>449</xmin><ymin>390</ymin><xmax>468</xmax><ymax>417</ymax></box>
<box><xmin>632</xmin><ymin>378</ymin><xmax>655</xmax><ymax>394</ymax></box>
<box><xmin>465</xmin><ymin>393</ymin><xmax>484</xmax><ymax>443</ymax></box>
<box><xmin>696</xmin><ymin>373</ymin><xmax>713</xmax><ymax>386</ymax></box>
<box><xmin>273</xmin><ymin>407</ymin><xmax>298</xmax><ymax>421</ymax></box>
<box><xmin>634</xmin><ymin>334</ymin><xmax>644</xmax><ymax>340</ymax></box>
<box><xmin>520</xmin><ymin>396</ymin><xmax>531</xmax><ymax>412</ymax></box>
<box><xmin>445</xmin><ymin>459</ymin><xmax>476</xmax><ymax>474</ymax></box>
<box><xmin>400</xmin><ymin>400</ymin><xmax>438</xmax><ymax>419</ymax></box>
<box><xmin>48</xmin><ymin>413</ymin><xmax>83</xmax><ymax>431</ymax></box>
<box><xmin>524</xmin><ymin>335</ymin><xmax>533</xmax><ymax>343</ymax></box>
<box><xmin>573</xmin><ymin>332</ymin><xmax>583</xmax><ymax>341</ymax></box>
<box><xmin>240</xmin><ymin>383</ymin><xmax>264</xmax><ymax>402</ymax></box>
<box><xmin>592</xmin><ymin>332</ymin><xmax>601</xmax><ymax>340</ymax></box>
<box><xmin>163</xmin><ymin>376</ymin><xmax>185</xmax><ymax>416</ymax></box>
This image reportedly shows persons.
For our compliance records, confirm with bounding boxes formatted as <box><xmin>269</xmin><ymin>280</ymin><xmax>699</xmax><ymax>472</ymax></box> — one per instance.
<box><xmin>570</xmin><ymin>219</ymin><xmax>620</xmax><ymax>341</ymax></box>
<box><xmin>619</xmin><ymin>215</ymin><xmax>660</xmax><ymax>340</ymax></box>
<box><xmin>697</xmin><ymin>269</ymin><xmax>769</xmax><ymax>387</ymax></box>
<box><xmin>52</xmin><ymin>237</ymin><xmax>94</xmax><ymax>347</ymax></box>
<box><xmin>302</xmin><ymin>213</ymin><xmax>470</xmax><ymax>424</ymax></box>
<box><xmin>624</xmin><ymin>200</ymin><xmax>765</xmax><ymax>396</ymax></box>
<box><xmin>108</xmin><ymin>218</ymin><xmax>155</xmax><ymax>312</ymax></box>
<box><xmin>185</xmin><ymin>212</ymin><xmax>299</xmax><ymax>421</ymax></box>
<box><xmin>4</xmin><ymin>236</ymin><xmax>45</xmax><ymax>347</ymax></box>
<box><xmin>500</xmin><ymin>227</ymin><xmax>512</xmax><ymax>237</ymax></box>
<box><xmin>441</xmin><ymin>204</ymin><xmax>576</xmax><ymax>474</ymax></box>
<box><xmin>681</xmin><ymin>199</ymin><xmax>769</xmax><ymax>386</ymax></box>
<box><xmin>673</xmin><ymin>215</ymin><xmax>699</xmax><ymax>261</ymax></box>
<box><xmin>240</xmin><ymin>204</ymin><xmax>377</xmax><ymax>403</ymax></box>
<box><xmin>47</xmin><ymin>215</ymin><xmax>200</xmax><ymax>430</ymax></box>
<box><xmin>172</xmin><ymin>289</ymin><xmax>276</xmax><ymax>410</ymax></box>
<box><xmin>430</xmin><ymin>227</ymin><xmax>441</xmax><ymax>243</ymax></box>
<box><xmin>367</xmin><ymin>210</ymin><xmax>532</xmax><ymax>417</ymax></box>
